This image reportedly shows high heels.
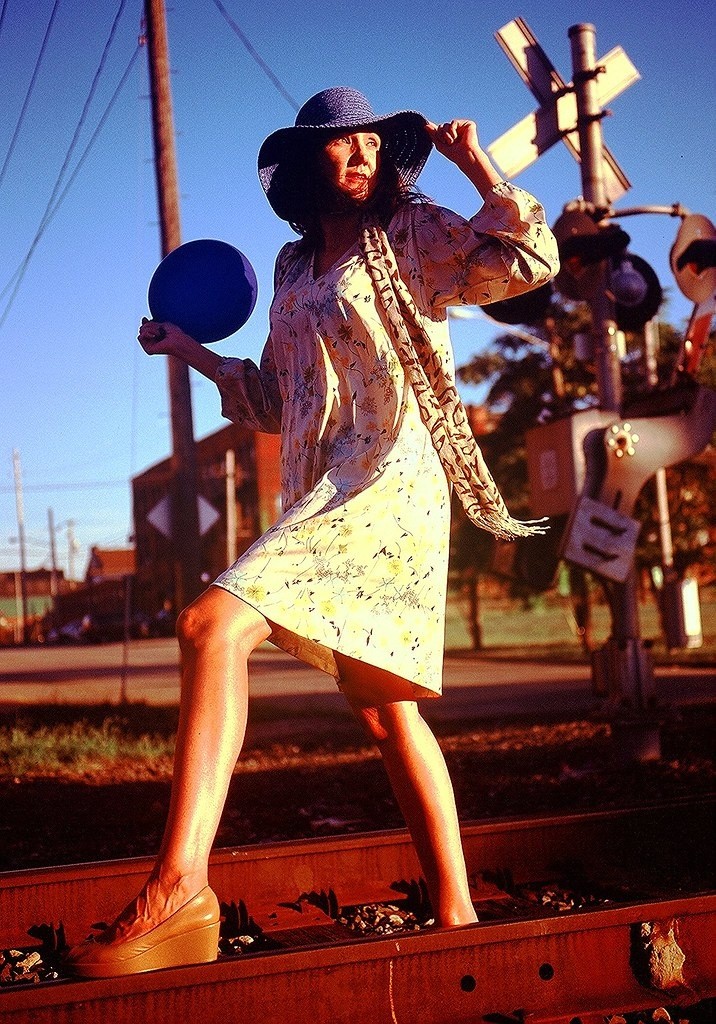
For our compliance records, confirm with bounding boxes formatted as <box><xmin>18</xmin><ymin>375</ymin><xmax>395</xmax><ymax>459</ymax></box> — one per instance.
<box><xmin>66</xmin><ymin>885</ymin><xmax>222</xmax><ymax>978</ymax></box>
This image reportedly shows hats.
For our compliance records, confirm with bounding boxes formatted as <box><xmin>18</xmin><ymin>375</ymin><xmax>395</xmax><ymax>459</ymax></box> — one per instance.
<box><xmin>257</xmin><ymin>86</ymin><xmax>435</xmax><ymax>230</ymax></box>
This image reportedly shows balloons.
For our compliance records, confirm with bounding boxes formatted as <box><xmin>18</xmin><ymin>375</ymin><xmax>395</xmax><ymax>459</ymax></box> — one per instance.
<box><xmin>148</xmin><ymin>238</ymin><xmax>258</xmax><ymax>345</ymax></box>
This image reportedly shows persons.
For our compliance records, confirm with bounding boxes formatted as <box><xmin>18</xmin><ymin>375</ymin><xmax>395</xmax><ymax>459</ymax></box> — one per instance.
<box><xmin>66</xmin><ymin>86</ymin><xmax>566</xmax><ymax>975</ymax></box>
